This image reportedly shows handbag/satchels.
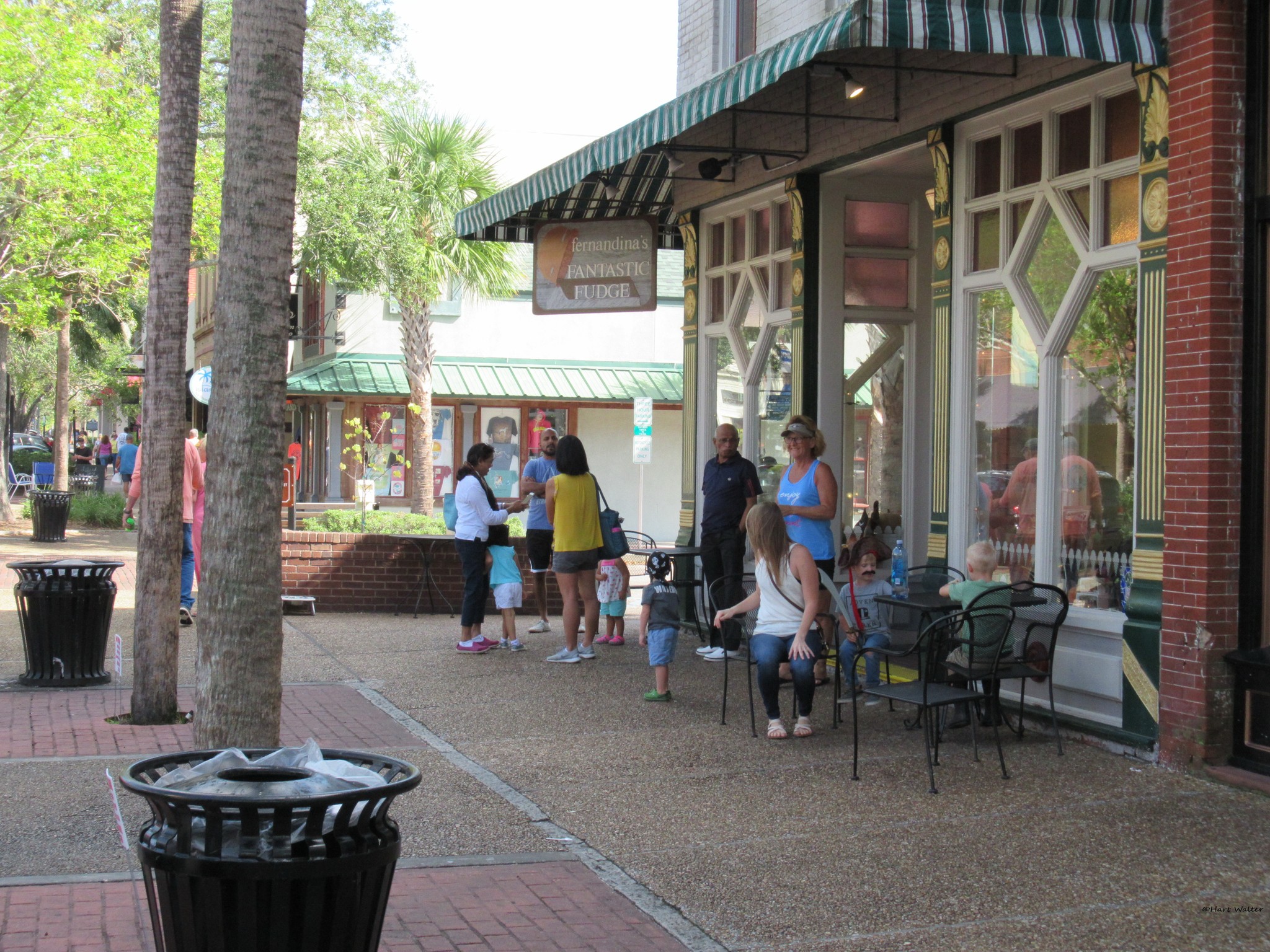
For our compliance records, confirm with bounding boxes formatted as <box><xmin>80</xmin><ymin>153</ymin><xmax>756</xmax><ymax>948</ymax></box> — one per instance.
<box><xmin>108</xmin><ymin>455</ymin><xmax>113</xmax><ymax>464</ymax></box>
<box><xmin>590</xmin><ymin>473</ymin><xmax>630</xmax><ymax>559</ymax></box>
<box><xmin>443</xmin><ymin>493</ymin><xmax>459</xmax><ymax>532</ymax></box>
<box><xmin>110</xmin><ymin>472</ymin><xmax>122</xmax><ymax>486</ymax></box>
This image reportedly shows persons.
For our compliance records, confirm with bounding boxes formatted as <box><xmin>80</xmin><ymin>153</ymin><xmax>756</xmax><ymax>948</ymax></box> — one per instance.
<box><xmin>986</xmin><ymin>436</ymin><xmax>1104</xmax><ymax>606</ymax></box>
<box><xmin>454</xmin><ymin>413</ymin><xmax>1013</xmax><ymax>740</ymax></box>
<box><xmin>23</xmin><ymin>425</ymin><xmax>208</xmax><ymax>626</ymax></box>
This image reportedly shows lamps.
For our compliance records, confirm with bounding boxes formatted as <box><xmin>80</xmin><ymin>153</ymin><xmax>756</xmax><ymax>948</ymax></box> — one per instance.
<box><xmin>601</xmin><ymin>177</ymin><xmax>620</xmax><ymax>200</ymax></box>
<box><xmin>662</xmin><ymin>147</ymin><xmax>685</xmax><ymax>174</ymax></box>
<box><xmin>839</xmin><ymin>69</ymin><xmax>867</xmax><ymax>99</ymax></box>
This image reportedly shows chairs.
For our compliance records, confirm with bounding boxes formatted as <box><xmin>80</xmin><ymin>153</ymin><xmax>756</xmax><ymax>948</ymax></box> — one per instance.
<box><xmin>625</xmin><ymin>530</ymin><xmax>1070</xmax><ymax>795</ymax></box>
<box><xmin>7</xmin><ymin>461</ymin><xmax>101</xmax><ymax>498</ymax></box>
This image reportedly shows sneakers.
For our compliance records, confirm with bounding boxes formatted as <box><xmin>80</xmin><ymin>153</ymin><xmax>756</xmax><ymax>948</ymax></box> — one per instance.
<box><xmin>577</xmin><ymin>643</ymin><xmax>596</xmax><ymax>660</ymax></box>
<box><xmin>529</xmin><ymin>620</ymin><xmax>550</xmax><ymax>633</ymax></box>
<box><xmin>546</xmin><ymin>648</ymin><xmax>581</xmax><ymax>663</ymax></box>
<box><xmin>578</xmin><ymin>624</ymin><xmax>585</xmax><ymax>632</ymax></box>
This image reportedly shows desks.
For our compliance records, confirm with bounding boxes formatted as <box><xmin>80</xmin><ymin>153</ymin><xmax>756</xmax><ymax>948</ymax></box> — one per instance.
<box><xmin>874</xmin><ymin>594</ymin><xmax>1048</xmax><ymax>747</ymax></box>
<box><xmin>389</xmin><ymin>535</ymin><xmax>455</xmax><ymax>619</ymax></box>
<box><xmin>628</xmin><ymin>547</ymin><xmax>709</xmax><ymax>643</ymax></box>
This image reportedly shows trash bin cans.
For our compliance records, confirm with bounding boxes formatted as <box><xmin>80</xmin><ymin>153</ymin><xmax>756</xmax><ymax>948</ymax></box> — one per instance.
<box><xmin>68</xmin><ymin>475</ymin><xmax>98</xmax><ymax>494</ymax></box>
<box><xmin>119</xmin><ymin>745</ymin><xmax>423</xmax><ymax>952</ymax></box>
<box><xmin>28</xmin><ymin>488</ymin><xmax>77</xmax><ymax>543</ymax></box>
<box><xmin>8</xmin><ymin>558</ymin><xmax>126</xmax><ymax>687</ymax></box>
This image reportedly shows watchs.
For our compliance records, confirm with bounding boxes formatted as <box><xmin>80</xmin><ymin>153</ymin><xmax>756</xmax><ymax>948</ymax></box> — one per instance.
<box><xmin>123</xmin><ymin>508</ymin><xmax>132</xmax><ymax>514</ymax></box>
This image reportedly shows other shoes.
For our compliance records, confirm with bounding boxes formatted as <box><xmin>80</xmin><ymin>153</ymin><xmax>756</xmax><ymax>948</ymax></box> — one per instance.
<box><xmin>838</xmin><ymin>688</ymin><xmax>865</xmax><ymax>701</ymax></box>
<box><xmin>179</xmin><ymin>605</ymin><xmax>193</xmax><ymax>625</ymax></box>
<box><xmin>950</xmin><ymin>713</ymin><xmax>969</xmax><ymax>727</ymax></box>
<box><xmin>696</xmin><ymin>644</ymin><xmax>720</xmax><ymax>656</ymax></box>
<box><xmin>455</xmin><ymin>640</ymin><xmax>490</xmax><ymax>654</ymax></box>
<box><xmin>981</xmin><ymin>716</ymin><xmax>1002</xmax><ymax>726</ymax></box>
<box><xmin>705</xmin><ymin>647</ymin><xmax>741</xmax><ymax>662</ymax></box>
<box><xmin>475</xmin><ymin>637</ymin><xmax>500</xmax><ymax>647</ymax></box>
<box><xmin>865</xmin><ymin>693</ymin><xmax>882</xmax><ymax>707</ymax></box>
<box><xmin>511</xmin><ymin>642</ymin><xmax>525</xmax><ymax>651</ymax></box>
<box><xmin>499</xmin><ymin>641</ymin><xmax>508</xmax><ymax>648</ymax></box>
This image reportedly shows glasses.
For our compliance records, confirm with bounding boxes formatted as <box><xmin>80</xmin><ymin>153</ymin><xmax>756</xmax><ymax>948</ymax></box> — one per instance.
<box><xmin>784</xmin><ymin>436</ymin><xmax>809</xmax><ymax>444</ymax></box>
<box><xmin>111</xmin><ymin>435</ymin><xmax>116</xmax><ymax>437</ymax></box>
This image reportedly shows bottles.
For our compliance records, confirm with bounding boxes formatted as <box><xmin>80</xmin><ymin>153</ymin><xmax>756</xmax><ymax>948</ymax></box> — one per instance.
<box><xmin>891</xmin><ymin>540</ymin><xmax>908</xmax><ymax>600</ymax></box>
<box><xmin>125</xmin><ymin>518</ymin><xmax>134</xmax><ymax>531</ymax></box>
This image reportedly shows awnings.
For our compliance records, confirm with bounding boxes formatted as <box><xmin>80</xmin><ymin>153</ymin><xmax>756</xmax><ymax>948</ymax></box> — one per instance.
<box><xmin>450</xmin><ymin>1</ymin><xmax>1174</xmax><ymax>240</ymax></box>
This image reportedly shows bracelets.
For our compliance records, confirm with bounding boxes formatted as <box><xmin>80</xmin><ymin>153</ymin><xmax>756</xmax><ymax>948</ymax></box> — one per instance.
<box><xmin>502</xmin><ymin>503</ymin><xmax>505</xmax><ymax>510</ymax></box>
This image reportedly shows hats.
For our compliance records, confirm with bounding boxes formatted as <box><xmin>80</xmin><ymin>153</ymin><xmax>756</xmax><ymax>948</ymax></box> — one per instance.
<box><xmin>647</xmin><ymin>551</ymin><xmax>671</xmax><ymax>583</ymax></box>
<box><xmin>77</xmin><ymin>438</ymin><xmax>84</xmax><ymax>442</ymax></box>
<box><xmin>780</xmin><ymin>424</ymin><xmax>815</xmax><ymax>437</ymax></box>
<box><xmin>839</xmin><ymin>537</ymin><xmax>893</xmax><ymax>566</ymax></box>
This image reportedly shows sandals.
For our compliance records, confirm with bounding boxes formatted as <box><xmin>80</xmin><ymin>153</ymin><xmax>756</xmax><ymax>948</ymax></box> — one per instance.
<box><xmin>815</xmin><ymin>676</ymin><xmax>830</xmax><ymax>687</ymax></box>
<box><xmin>609</xmin><ymin>636</ymin><xmax>625</xmax><ymax>645</ymax></box>
<box><xmin>779</xmin><ymin>677</ymin><xmax>793</xmax><ymax>683</ymax></box>
<box><xmin>644</xmin><ymin>688</ymin><xmax>672</xmax><ymax>701</ymax></box>
<box><xmin>767</xmin><ymin>719</ymin><xmax>787</xmax><ymax>739</ymax></box>
<box><xmin>793</xmin><ymin>714</ymin><xmax>812</xmax><ymax>737</ymax></box>
<box><xmin>595</xmin><ymin>634</ymin><xmax>614</xmax><ymax>644</ymax></box>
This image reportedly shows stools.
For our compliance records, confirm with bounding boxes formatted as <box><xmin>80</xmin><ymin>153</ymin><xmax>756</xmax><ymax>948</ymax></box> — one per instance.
<box><xmin>281</xmin><ymin>595</ymin><xmax>316</xmax><ymax>616</ymax></box>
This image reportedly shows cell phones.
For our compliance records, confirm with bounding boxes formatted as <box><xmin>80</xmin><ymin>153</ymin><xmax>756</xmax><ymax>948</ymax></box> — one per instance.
<box><xmin>522</xmin><ymin>492</ymin><xmax>534</xmax><ymax>504</ymax></box>
